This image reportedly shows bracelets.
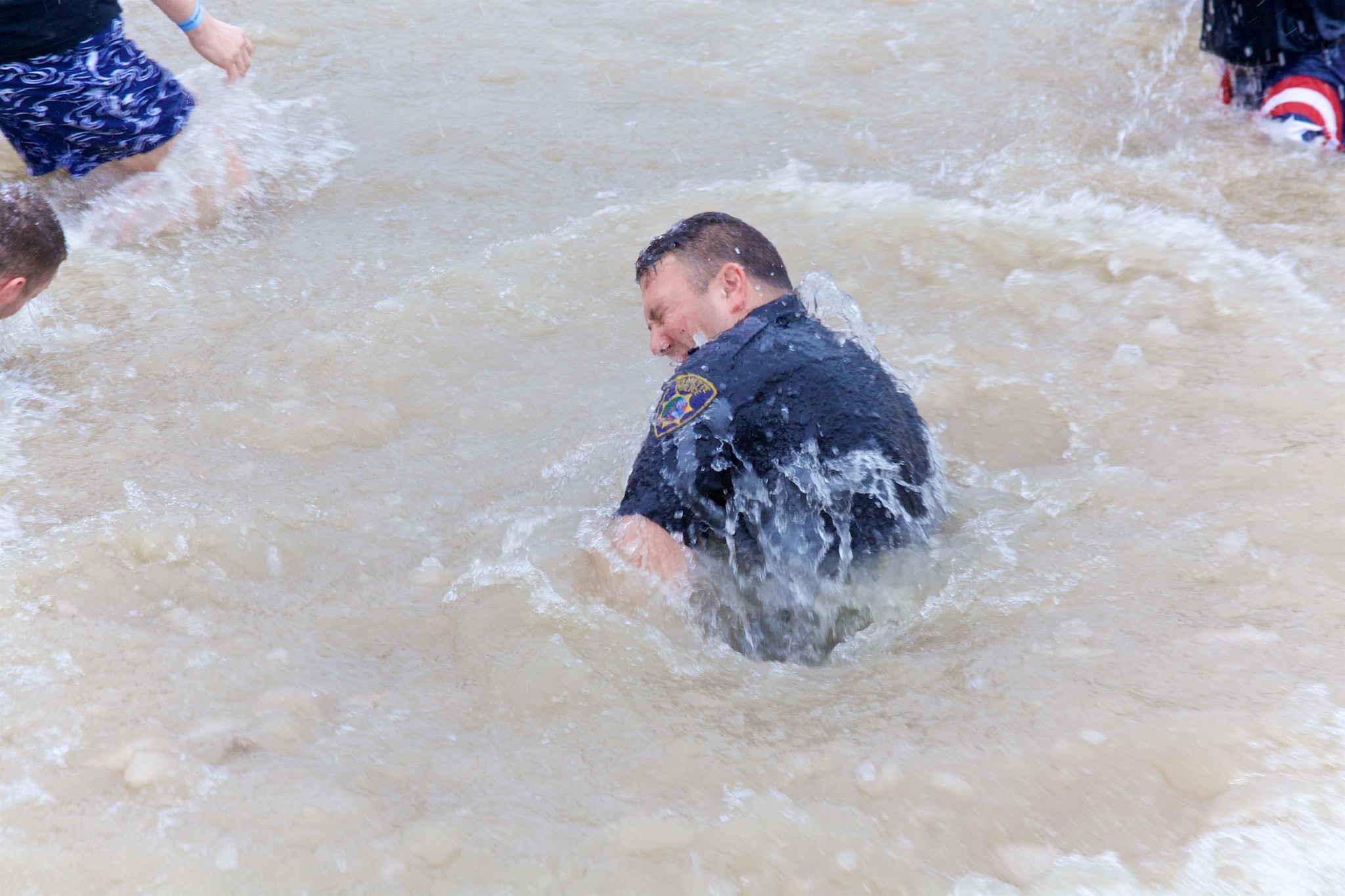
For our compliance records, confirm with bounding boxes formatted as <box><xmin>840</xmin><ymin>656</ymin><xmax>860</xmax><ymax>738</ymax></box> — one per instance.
<box><xmin>177</xmin><ymin>1</ymin><xmax>205</xmax><ymax>36</ymax></box>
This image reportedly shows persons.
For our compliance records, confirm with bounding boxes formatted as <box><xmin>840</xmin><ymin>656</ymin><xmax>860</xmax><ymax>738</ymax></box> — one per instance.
<box><xmin>0</xmin><ymin>2</ymin><xmax>260</xmax><ymax>207</ymax></box>
<box><xmin>593</xmin><ymin>209</ymin><xmax>942</xmax><ymax>670</ymax></box>
<box><xmin>0</xmin><ymin>183</ymin><xmax>71</xmax><ymax>323</ymax></box>
